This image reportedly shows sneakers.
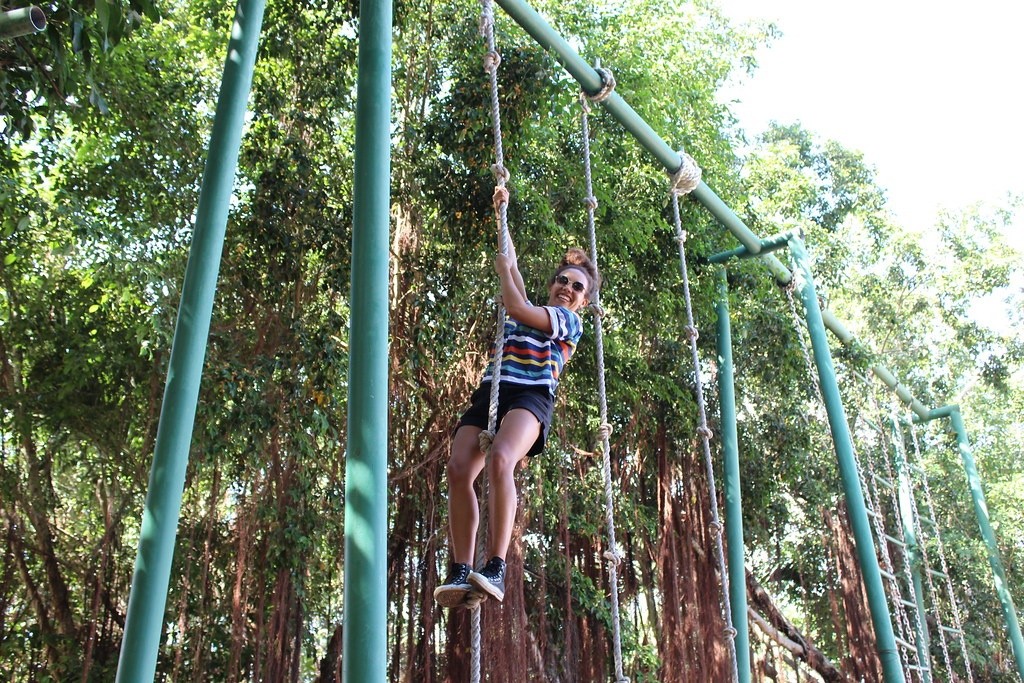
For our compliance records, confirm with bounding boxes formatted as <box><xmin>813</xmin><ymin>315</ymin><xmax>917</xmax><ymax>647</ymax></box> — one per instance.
<box><xmin>466</xmin><ymin>556</ymin><xmax>506</xmax><ymax>602</ymax></box>
<box><xmin>434</xmin><ymin>563</ymin><xmax>473</xmax><ymax>607</ymax></box>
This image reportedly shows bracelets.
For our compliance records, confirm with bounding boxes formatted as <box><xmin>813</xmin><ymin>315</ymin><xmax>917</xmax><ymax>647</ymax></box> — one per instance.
<box><xmin>496</xmin><ymin>218</ymin><xmax>501</xmax><ymax>220</ymax></box>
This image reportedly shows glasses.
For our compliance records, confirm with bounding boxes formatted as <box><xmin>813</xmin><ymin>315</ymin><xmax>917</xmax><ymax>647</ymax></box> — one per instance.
<box><xmin>557</xmin><ymin>275</ymin><xmax>586</xmax><ymax>292</ymax></box>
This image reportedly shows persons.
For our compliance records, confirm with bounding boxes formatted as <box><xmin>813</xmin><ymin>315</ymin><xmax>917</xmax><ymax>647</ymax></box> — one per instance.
<box><xmin>434</xmin><ymin>185</ymin><xmax>599</xmax><ymax>608</ymax></box>
